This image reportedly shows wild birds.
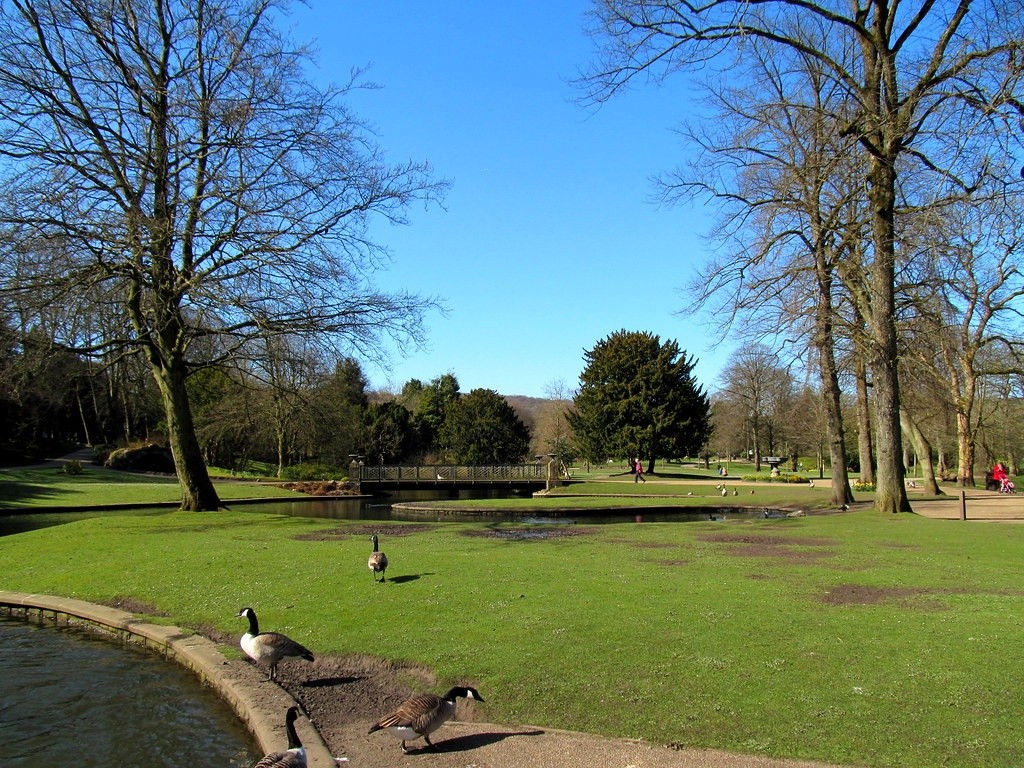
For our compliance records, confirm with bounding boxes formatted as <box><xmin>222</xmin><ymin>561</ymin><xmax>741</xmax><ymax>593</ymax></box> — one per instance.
<box><xmin>809</xmin><ymin>479</ymin><xmax>815</xmax><ymax>490</ymax></box>
<box><xmin>254</xmin><ymin>705</ymin><xmax>306</xmax><ymax>768</ymax></box>
<box><xmin>688</xmin><ymin>491</ymin><xmax>693</xmax><ymax>495</ymax></box>
<box><xmin>715</xmin><ymin>483</ymin><xmax>738</xmax><ymax>497</ymax></box>
<box><xmin>437</xmin><ymin>474</ymin><xmax>444</xmax><ymax>479</ymax></box>
<box><xmin>367</xmin><ymin>686</ymin><xmax>485</xmax><ymax>754</ymax></box>
<box><xmin>708</xmin><ymin>512</ymin><xmax>716</xmax><ymax>521</ymax></box>
<box><xmin>838</xmin><ymin>504</ymin><xmax>849</xmax><ymax>512</ymax></box>
<box><xmin>234</xmin><ymin>607</ymin><xmax>314</xmax><ymax>683</ymax></box>
<box><xmin>764</xmin><ymin>508</ymin><xmax>768</xmax><ymax>519</ymax></box>
<box><xmin>367</xmin><ymin>535</ymin><xmax>388</xmax><ymax>583</ymax></box>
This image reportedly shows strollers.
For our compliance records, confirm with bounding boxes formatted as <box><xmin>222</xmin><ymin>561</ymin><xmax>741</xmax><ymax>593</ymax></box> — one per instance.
<box><xmin>998</xmin><ymin>472</ymin><xmax>1017</xmax><ymax>494</ymax></box>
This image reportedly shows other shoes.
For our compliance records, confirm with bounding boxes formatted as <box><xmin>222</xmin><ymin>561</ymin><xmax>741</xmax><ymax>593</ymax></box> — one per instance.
<box><xmin>986</xmin><ymin>486</ymin><xmax>989</xmax><ymax>490</ymax></box>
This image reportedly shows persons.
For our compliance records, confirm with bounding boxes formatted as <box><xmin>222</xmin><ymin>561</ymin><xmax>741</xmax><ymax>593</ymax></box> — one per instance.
<box><xmin>635</xmin><ymin>459</ymin><xmax>646</xmax><ymax>483</ymax></box>
<box><xmin>992</xmin><ymin>462</ymin><xmax>1006</xmax><ymax>491</ymax></box>
<box><xmin>722</xmin><ymin>467</ymin><xmax>728</xmax><ymax>476</ymax></box>
